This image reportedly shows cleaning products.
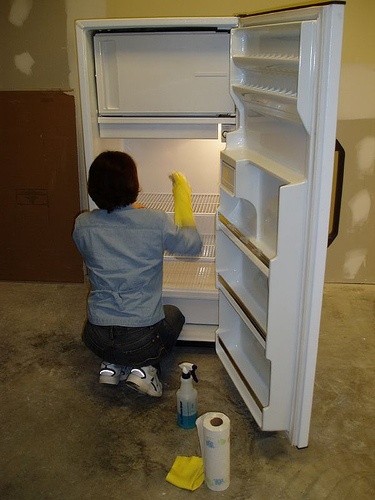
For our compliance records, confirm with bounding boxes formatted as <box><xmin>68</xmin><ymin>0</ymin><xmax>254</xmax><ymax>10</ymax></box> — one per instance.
<box><xmin>177</xmin><ymin>362</ymin><xmax>198</xmax><ymax>430</ymax></box>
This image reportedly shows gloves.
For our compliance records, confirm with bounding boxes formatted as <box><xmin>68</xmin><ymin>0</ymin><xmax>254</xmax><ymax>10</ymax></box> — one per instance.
<box><xmin>165</xmin><ymin>455</ymin><xmax>205</xmax><ymax>491</ymax></box>
<box><xmin>168</xmin><ymin>169</ymin><xmax>196</xmax><ymax>227</ymax></box>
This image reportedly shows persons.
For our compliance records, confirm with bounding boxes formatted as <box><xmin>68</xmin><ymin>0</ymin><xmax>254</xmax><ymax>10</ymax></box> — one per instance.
<box><xmin>73</xmin><ymin>150</ymin><xmax>203</xmax><ymax>397</ymax></box>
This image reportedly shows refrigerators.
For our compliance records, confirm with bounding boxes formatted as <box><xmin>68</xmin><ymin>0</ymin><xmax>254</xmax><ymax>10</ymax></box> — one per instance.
<box><xmin>74</xmin><ymin>0</ymin><xmax>347</xmax><ymax>450</ymax></box>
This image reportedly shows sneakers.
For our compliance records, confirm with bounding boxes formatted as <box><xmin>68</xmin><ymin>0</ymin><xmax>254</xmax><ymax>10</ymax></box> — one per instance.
<box><xmin>125</xmin><ymin>364</ymin><xmax>163</xmax><ymax>398</ymax></box>
<box><xmin>98</xmin><ymin>360</ymin><xmax>133</xmax><ymax>385</ymax></box>
